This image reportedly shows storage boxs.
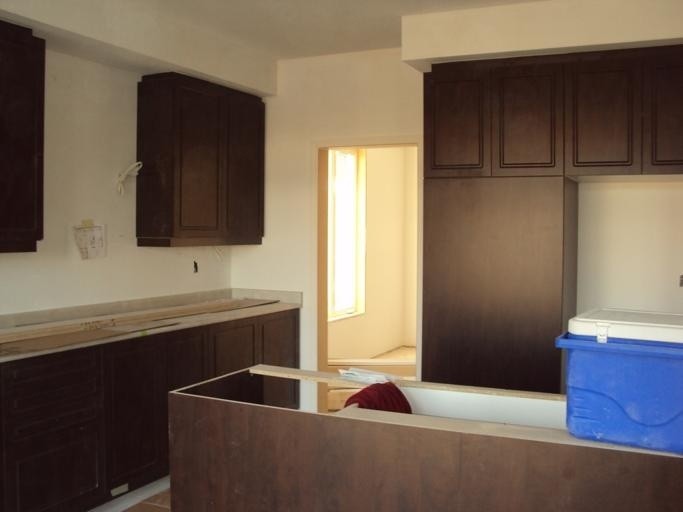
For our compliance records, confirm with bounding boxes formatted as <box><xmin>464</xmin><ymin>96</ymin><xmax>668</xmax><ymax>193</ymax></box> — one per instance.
<box><xmin>552</xmin><ymin>306</ymin><xmax>683</xmax><ymax>456</ymax></box>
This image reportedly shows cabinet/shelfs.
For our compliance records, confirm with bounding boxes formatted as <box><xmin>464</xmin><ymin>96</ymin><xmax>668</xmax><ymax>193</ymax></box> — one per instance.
<box><xmin>563</xmin><ymin>44</ymin><xmax>682</xmax><ymax>176</ymax></box>
<box><xmin>134</xmin><ymin>72</ymin><xmax>266</xmax><ymax>248</ymax></box>
<box><xmin>424</xmin><ymin>56</ymin><xmax>564</xmax><ymax>176</ymax></box>
<box><xmin>1</xmin><ymin>19</ymin><xmax>47</xmax><ymax>253</ymax></box>
<box><xmin>106</xmin><ymin>324</ymin><xmax>207</xmax><ymax>503</ymax></box>
<box><xmin>421</xmin><ymin>175</ymin><xmax>578</xmax><ymax>395</ymax></box>
<box><xmin>209</xmin><ymin>308</ymin><xmax>299</xmax><ymax>408</ymax></box>
<box><xmin>0</xmin><ymin>345</ymin><xmax>107</xmax><ymax>512</ymax></box>
